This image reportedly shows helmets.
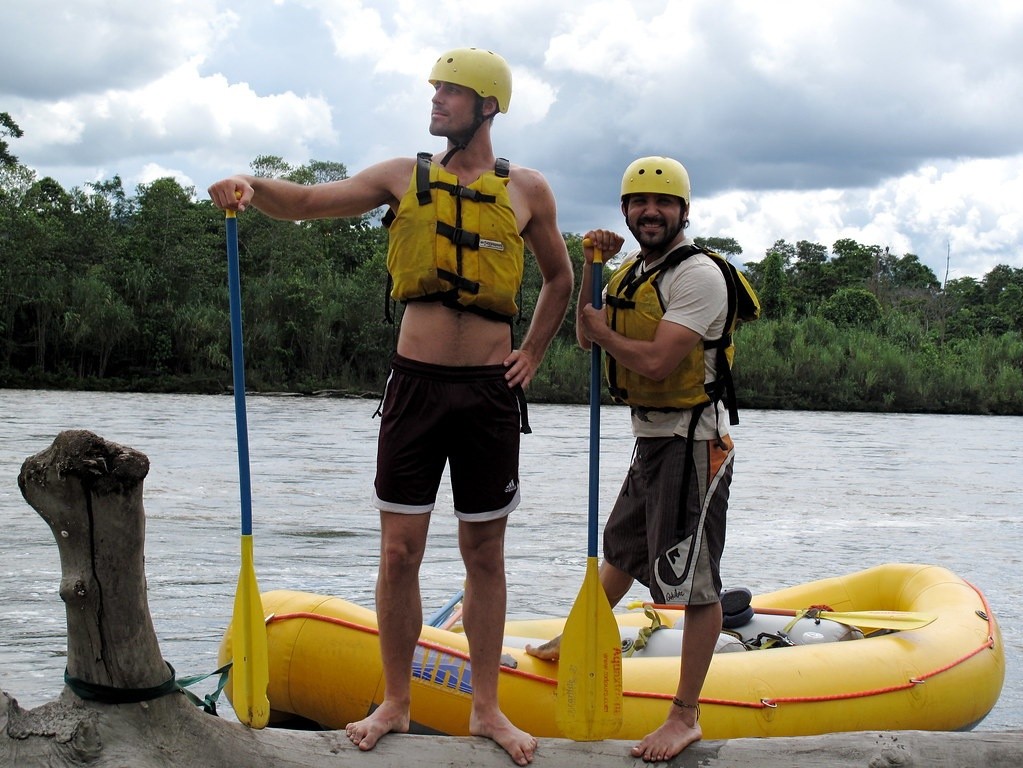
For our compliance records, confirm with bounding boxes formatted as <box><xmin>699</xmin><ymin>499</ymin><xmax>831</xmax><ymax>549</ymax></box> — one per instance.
<box><xmin>428</xmin><ymin>47</ymin><xmax>512</xmax><ymax>114</ymax></box>
<box><xmin>620</xmin><ymin>156</ymin><xmax>690</xmax><ymax>217</ymax></box>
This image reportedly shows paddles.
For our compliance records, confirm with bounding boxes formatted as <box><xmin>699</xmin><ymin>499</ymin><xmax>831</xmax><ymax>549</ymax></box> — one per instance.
<box><xmin>224</xmin><ymin>194</ymin><xmax>270</xmax><ymax>728</ymax></box>
<box><xmin>557</xmin><ymin>241</ymin><xmax>624</xmax><ymax>741</ymax></box>
<box><xmin>628</xmin><ymin>600</ymin><xmax>940</xmax><ymax>631</ymax></box>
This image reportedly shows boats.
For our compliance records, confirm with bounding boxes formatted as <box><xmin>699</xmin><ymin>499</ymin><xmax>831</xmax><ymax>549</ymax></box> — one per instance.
<box><xmin>260</xmin><ymin>563</ymin><xmax>1007</xmax><ymax>747</ymax></box>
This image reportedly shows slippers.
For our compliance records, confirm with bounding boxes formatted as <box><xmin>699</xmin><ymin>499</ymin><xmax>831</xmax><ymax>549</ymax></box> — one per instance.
<box><xmin>719</xmin><ymin>586</ymin><xmax>754</xmax><ymax>629</ymax></box>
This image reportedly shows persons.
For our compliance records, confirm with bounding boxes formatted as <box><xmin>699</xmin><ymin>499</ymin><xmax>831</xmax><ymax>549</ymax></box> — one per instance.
<box><xmin>209</xmin><ymin>49</ymin><xmax>573</xmax><ymax>766</ymax></box>
<box><xmin>526</xmin><ymin>156</ymin><xmax>734</xmax><ymax>764</ymax></box>
